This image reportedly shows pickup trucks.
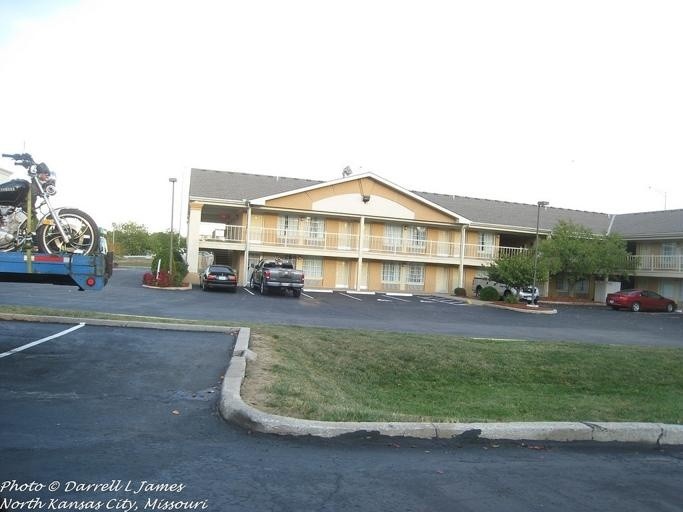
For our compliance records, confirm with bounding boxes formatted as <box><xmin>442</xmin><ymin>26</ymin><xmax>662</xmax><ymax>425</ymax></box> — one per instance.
<box><xmin>248</xmin><ymin>257</ymin><xmax>306</xmax><ymax>298</ymax></box>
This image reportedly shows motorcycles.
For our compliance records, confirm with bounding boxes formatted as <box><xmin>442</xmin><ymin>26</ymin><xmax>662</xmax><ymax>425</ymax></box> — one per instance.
<box><xmin>1</xmin><ymin>149</ymin><xmax>101</xmax><ymax>258</ymax></box>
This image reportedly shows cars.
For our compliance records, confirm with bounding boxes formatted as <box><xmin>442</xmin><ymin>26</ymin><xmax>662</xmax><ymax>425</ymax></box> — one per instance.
<box><xmin>470</xmin><ymin>271</ymin><xmax>541</xmax><ymax>306</ymax></box>
<box><xmin>605</xmin><ymin>287</ymin><xmax>678</xmax><ymax>314</ymax></box>
<box><xmin>199</xmin><ymin>263</ymin><xmax>238</xmax><ymax>293</ymax></box>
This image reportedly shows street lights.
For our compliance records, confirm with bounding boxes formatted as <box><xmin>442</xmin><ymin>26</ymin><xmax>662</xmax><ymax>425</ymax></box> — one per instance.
<box><xmin>530</xmin><ymin>200</ymin><xmax>550</xmax><ymax>307</ymax></box>
<box><xmin>168</xmin><ymin>176</ymin><xmax>179</xmax><ymax>281</ymax></box>
